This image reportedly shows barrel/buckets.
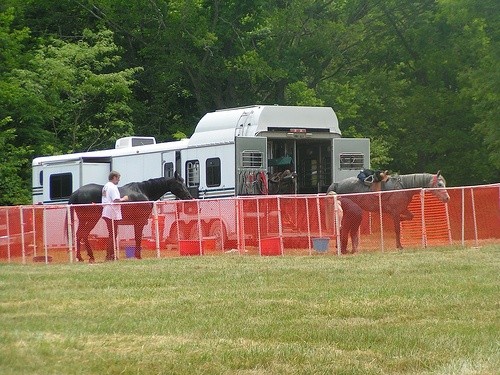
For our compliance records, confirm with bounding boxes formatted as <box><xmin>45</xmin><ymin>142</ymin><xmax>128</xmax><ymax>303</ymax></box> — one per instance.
<box><xmin>311</xmin><ymin>238</ymin><xmax>330</xmax><ymax>250</ymax></box>
<box><xmin>262</xmin><ymin>237</ymin><xmax>283</xmax><ymax>255</ymax></box>
<box><xmin>180</xmin><ymin>240</ymin><xmax>204</xmax><ymax>255</ymax></box>
<box><xmin>126</xmin><ymin>246</ymin><xmax>136</xmax><ymax>258</ymax></box>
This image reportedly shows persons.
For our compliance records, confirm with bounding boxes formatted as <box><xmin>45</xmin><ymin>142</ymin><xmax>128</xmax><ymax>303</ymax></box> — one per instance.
<box><xmin>325</xmin><ymin>191</ymin><xmax>364</xmax><ymax>254</ymax></box>
<box><xmin>101</xmin><ymin>171</ymin><xmax>128</xmax><ymax>260</ymax></box>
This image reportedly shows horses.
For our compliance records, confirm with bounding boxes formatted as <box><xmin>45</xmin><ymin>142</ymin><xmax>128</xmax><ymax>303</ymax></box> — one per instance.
<box><xmin>63</xmin><ymin>169</ymin><xmax>193</xmax><ymax>263</ymax></box>
<box><xmin>325</xmin><ymin>170</ymin><xmax>451</xmax><ymax>254</ymax></box>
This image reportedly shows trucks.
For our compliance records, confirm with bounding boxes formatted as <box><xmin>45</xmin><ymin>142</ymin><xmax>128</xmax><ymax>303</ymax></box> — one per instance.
<box><xmin>32</xmin><ymin>104</ymin><xmax>370</xmax><ymax>248</ymax></box>
